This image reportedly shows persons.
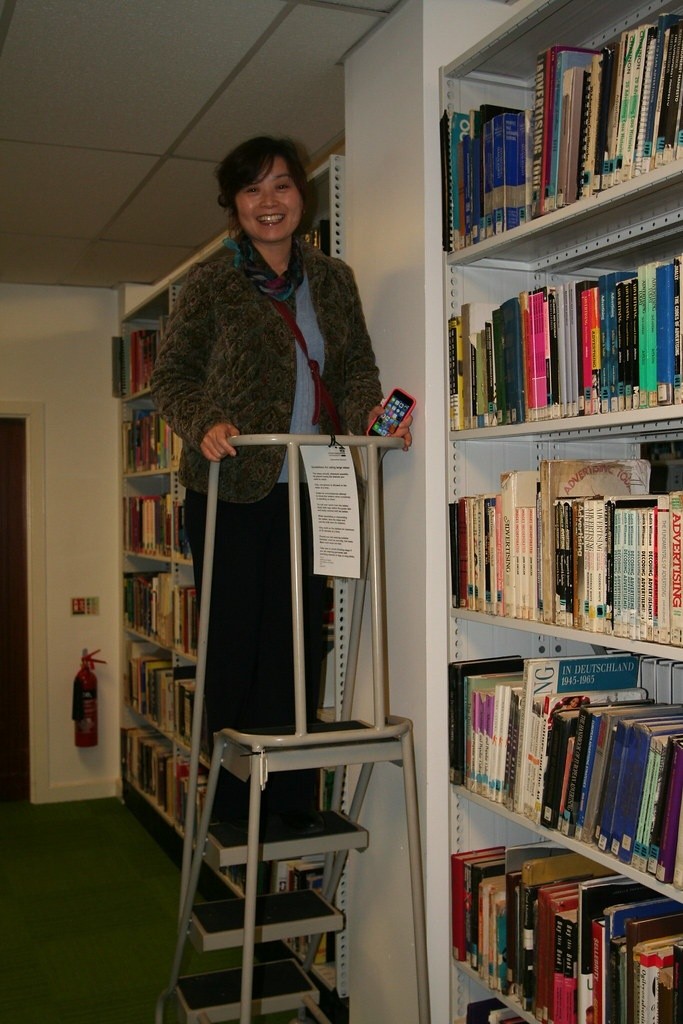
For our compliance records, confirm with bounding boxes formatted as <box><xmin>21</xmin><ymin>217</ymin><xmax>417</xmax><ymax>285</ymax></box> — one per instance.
<box><xmin>148</xmin><ymin>137</ymin><xmax>412</xmax><ymax>836</ymax></box>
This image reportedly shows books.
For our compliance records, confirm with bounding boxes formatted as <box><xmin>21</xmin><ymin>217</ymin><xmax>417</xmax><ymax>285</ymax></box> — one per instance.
<box><xmin>449</xmin><ymin>256</ymin><xmax>683</xmax><ymax>431</ymax></box>
<box><xmin>452</xmin><ymin>841</ymin><xmax>683</xmax><ymax>1024</ymax></box>
<box><xmin>441</xmin><ymin>14</ymin><xmax>683</xmax><ymax>251</ymax></box>
<box><xmin>450</xmin><ymin>458</ymin><xmax>683</xmax><ymax>646</ymax></box>
<box><xmin>122</xmin><ymin>221</ymin><xmax>335</xmax><ymax>963</ymax></box>
<box><xmin>449</xmin><ymin>653</ymin><xmax>683</xmax><ymax>891</ymax></box>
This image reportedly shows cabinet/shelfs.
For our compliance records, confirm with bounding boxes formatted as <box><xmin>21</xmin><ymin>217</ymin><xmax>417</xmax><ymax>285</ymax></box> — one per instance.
<box><xmin>437</xmin><ymin>0</ymin><xmax>683</xmax><ymax>1024</ymax></box>
<box><xmin>117</xmin><ymin>155</ymin><xmax>347</xmax><ymax>999</ymax></box>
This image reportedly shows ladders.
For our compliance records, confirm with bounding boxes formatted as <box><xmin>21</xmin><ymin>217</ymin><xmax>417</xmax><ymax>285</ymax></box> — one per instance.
<box><xmin>152</xmin><ymin>430</ymin><xmax>435</xmax><ymax>1024</ymax></box>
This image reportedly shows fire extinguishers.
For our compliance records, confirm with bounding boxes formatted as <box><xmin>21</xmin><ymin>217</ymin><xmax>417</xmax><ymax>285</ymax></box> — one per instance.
<box><xmin>73</xmin><ymin>648</ymin><xmax>107</xmax><ymax>748</ymax></box>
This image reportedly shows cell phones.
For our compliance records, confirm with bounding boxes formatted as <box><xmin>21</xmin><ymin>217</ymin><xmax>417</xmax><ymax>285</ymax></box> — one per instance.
<box><xmin>367</xmin><ymin>386</ymin><xmax>416</xmax><ymax>437</ymax></box>
